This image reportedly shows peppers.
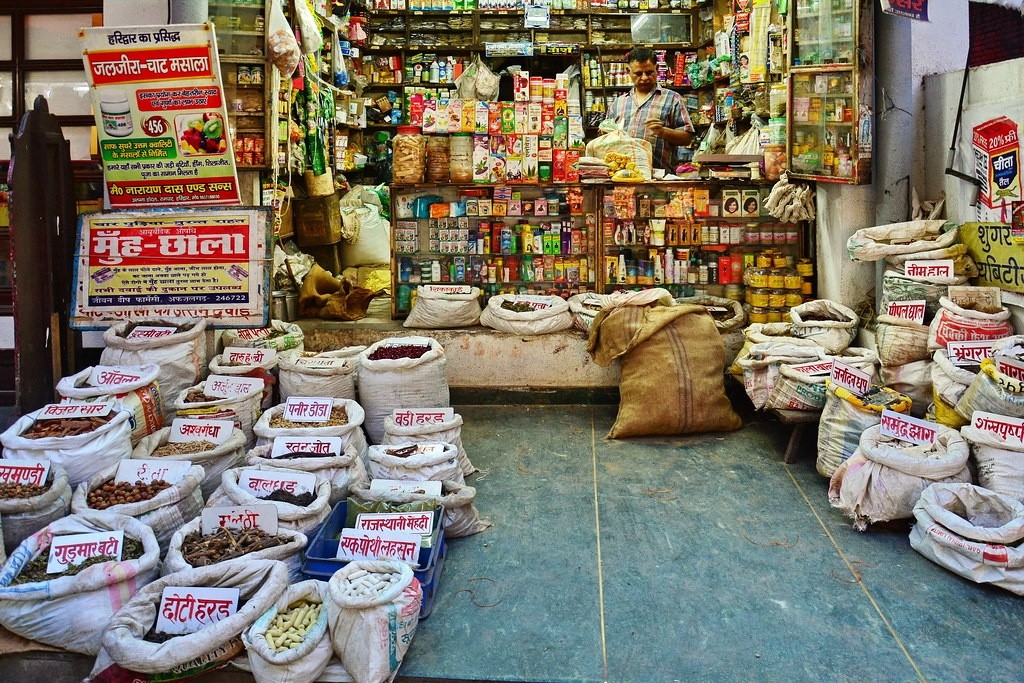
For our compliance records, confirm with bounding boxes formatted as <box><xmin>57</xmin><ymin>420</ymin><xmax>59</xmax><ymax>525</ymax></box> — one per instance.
<box><xmin>368</xmin><ymin>344</ymin><xmax>431</xmax><ymax>358</ymax></box>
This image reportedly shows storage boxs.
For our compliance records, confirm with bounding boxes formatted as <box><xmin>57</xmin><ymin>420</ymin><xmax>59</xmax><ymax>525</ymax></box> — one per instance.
<box><xmin>665</xmin><ymin>223</ymin><xmax>700</xmax><ymax>245</ymax></box>
<box><xmin>640</xmin><ymin>200</ymin><xmax>667</xmax><ymax>218</ymax></box>
<box><xmin>452</xmin><ymin>255</ymin><xmax>465</xmax><ymax>283</ymax></box>
<box><xmin>301</xmin><ymin>501</ymin><xmax>447</xmax><ymax>620</ymax></box>
<box><xmin>337</xmin><ymin>64</ymin><xmax>596</xmax><ymax>283</ymax></box>
<box><xmin>718</xmin><ymin>252</ymin><xmax>755</xmax><ymax>285</ymax></box>
<box><xmin>718</xmin><ymin>221</ymin><xmax>745</xmax><ymax>244</ymax></box>
<box><xmin>720</xmin><ymin>189</ymin><xmax>770</xmax><ymax>218</ymax></box>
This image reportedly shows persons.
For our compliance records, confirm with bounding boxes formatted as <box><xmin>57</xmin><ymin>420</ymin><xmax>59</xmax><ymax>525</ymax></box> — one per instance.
<box><xmin>739</xmin><ymin>56</ymin><xmax>750</xmax><ymax>74</ymax></box>
<box><xmin>724</xmin><ymin>198</ymin><xmax>758</xmax><ymax>217</ymax></box>
<box><xmin>601</xmin><ymin>48</ymin><xmax>695</xmax><ymax>176</ymax></box>
<box><xmin>641</xmin><ymin>225</ymin><xmax>650</xmax><ymax>245</ymax></box>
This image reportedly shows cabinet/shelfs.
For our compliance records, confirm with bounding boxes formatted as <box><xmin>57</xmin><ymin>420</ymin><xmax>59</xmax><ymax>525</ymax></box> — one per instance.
<box><xmin>210</xmin><ymin>1</ymin><xmax>273</xmax><ymax>169</ymax></box>
<box><xmin>278</xmin><ymin>1</ymin><xmax>781</xmax><ymax>173</ymax></box>
<box><xmin>787</xmin><ymin>0</ymin><xmax>873</xmax><ymax>185</ymax></box>
<box><xmin>600</xmin><ymin>180</ymin><xmax>804</xmax><ymax>306</ymax></box>
<box><xmin>389</xmin><ymin>180</ymin><xmax>599</xmax><ymax>320</ymax></box>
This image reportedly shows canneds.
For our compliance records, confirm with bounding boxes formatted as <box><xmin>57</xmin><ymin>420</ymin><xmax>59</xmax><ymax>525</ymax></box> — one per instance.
<box><xmin>237</xmin><ymin>66</ymin><xmax>264</xmax><ymax>84</ymax></box>
<box><xmin>746</xmin><ymin>221</ymin><xmax>799</xmax><ymax>244</ymax></box>
<box><xmin>602</xmin><ymin>63</ymin><xmax>635</xmax><ymax>86</ymax></box>
<box><xmin>744</xmin><ymin>252</ymin><xmax>814</xmax><ymax>324</ymax></box>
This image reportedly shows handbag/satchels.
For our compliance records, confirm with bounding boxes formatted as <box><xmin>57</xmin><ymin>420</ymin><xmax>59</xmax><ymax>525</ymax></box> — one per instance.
<box><xmin>292</xmin><ymin>0</ymin><xmax>323</xmax><ymax>55</ymax></box>
<box><xmin>452</xmin><ymin>53</ymin><xmax>504</xmax><ymax>101</ymax></box>
<box><xmin>692</xmin><ymin>123</ymin><xmax>761</xmax><ymax>166</ymax></box>
<box><xmin>332</xmin><ymin>28</ymin><xmax>347</xmax><ymax>88</ymax></box>
<box><xmin>269</xmin><ymin>0</ymin><xmax>301</xmax><ymax>79</ymax></box>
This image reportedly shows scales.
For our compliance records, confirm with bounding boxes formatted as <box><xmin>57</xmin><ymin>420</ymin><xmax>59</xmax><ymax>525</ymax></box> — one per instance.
<box><xmin>696</xmin><ymin>153</ymin><xmax>762</xmax><ymax>181</ymax></box>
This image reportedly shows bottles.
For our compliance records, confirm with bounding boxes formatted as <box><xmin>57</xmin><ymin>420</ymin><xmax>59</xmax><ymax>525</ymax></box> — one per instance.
<box><xmin>488</xmin><ymin>256</ymin><xmax>518</xmax><ymax>284</ymax></box>
<box><xmin>463</xmin><ymin>61</ymin><xmax>470</xmax><ymax>71</ymax></box>
<box><xmin>238</xmin><ymin>66</ymin><xmax>264</xmax><ymax>84</ymax></box>
<box><xmin>770</xmin><ymin>85</ymin><xmax>786</xmax><ymax>118</ymax></box>
<box><xmin>450</xmin><ymin>132</ymin><xmax>473</xmax><ymax>183</ymax></box>
<box><xmin>725</xmin><ymin>284</ymin><xmax>742</xmax><ymax>301</ymax></box>
<box><xmin>483</xmin><ymin>293</ymin><xmax>490</xmax><ymax>310</ymax></box>
<box><xmin>405</xmin><ymin>61</ymin><xmax>413</xmax><ymax>82</ymax></box>
<box><xmin>421</xmin><ymin>63</ymin><xmax>429</xmax><ymax>83</ymax></box>
<box><xmin>617</xmin><ymin>254</ymin><xmax>626</xmax><ymax>283</ymax></box>
<box><xmin>100</xmin><ymin>87</ymin><xmax>134</xmax><ymax>136</ymax></box>
<box><xmin>396</xmin><ymin>283</ymin><xmax>411</xmax><ymax>313</ymax></box>
<box><xmin>445</xmin><ymin>61</ymin><xmax>453</xmax><ymax>83</ymax></box>
<box><xmin>401</xmin><ymin>257</ymin><xmax>413</xmax><ymax>281</ymax></box>
<box><xmin>665</xmin><ymin>249</ymin><xmax>674</xmax><ymax>284</ymax></box>
<box><xmin>687</xmin><ymin>248</ymin><xmax>719</xmax><ymax>284</ymax></box>
<box><xmin>426</xmin><ymin>133</ymin><xmax>451</xmax><ymax>183</ymax></box>
<box><xmin>701</xmin><ymin>226</ymin><xmax>719</xmax><ymax>245</ymax></box>
<box><xmin>429</xmin><ymin>58</ymin><xmax>439</xmax><ymax>83</ymax></box>
<box><xmin>465</xmin><ymin>268</ymin><xmax>473</xmax><ymax>283</ymax></box>
<box><xmin>755</xmin><ymin>83</ymin><xmax>770</xmax><ymax>116</ymax></box>
<box><xmin>431</xmin><ymin>261</ymin><xmax>441</xmax><ymax>282</ymax></box>
<box><xmin>392</xmin><ymin>126</ymin><xmax>425</xmax><ymax>184</ymax></box>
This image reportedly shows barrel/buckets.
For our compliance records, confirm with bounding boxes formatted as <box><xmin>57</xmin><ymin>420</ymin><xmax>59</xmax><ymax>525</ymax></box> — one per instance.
<box><xmin>272</xmin><ymin>291</ymin><xmax>300</xmax><ymax>321</ymax></box>
<box><xmin>272</xmin><ymin>296</ymin><xmax>288</xmax><ymax>322</ymax></box>
